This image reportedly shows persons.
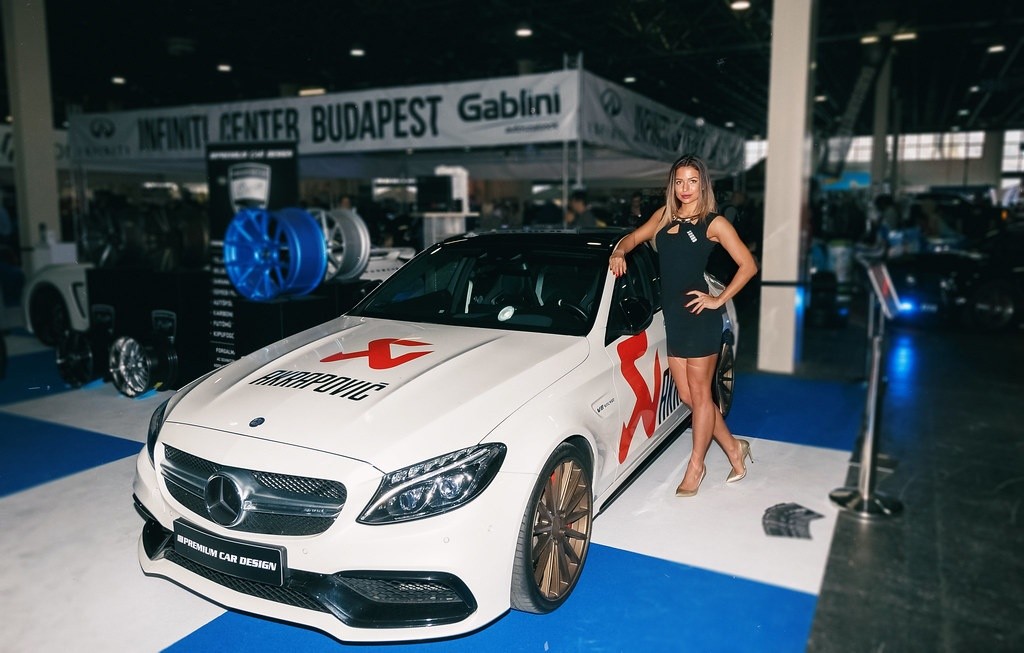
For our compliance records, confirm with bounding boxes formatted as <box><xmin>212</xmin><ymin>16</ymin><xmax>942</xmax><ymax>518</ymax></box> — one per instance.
<box><xmin>608</xmin><ymin>153</ymin><xmax>755</xmax><ymax>497</ymax></box>
<box><xmin>567</xmin><ymin>190</ymin><xmax>651</xmax><ymax>248</ymax></box>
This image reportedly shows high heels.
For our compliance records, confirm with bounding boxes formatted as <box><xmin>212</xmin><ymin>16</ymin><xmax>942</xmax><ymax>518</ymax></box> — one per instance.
<box><xmin>726</xmin><ymin>440</ymin><xmax>754</xmax><ymax>482</ymax></box>
<box><xmin>675</xmin><ymin>463</ymin><xmax>706</xmax><ymax>497</ymax></box>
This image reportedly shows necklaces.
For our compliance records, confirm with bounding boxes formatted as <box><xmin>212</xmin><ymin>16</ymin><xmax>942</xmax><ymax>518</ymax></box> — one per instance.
<box><xmin>670</xmin><ymin>204</ymin><xmax>702</xmax><ymax>224</ymax></box>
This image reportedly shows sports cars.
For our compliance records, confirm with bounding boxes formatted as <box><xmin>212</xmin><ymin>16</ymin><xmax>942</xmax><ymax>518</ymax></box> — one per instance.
<box><xmin>131</xmin><ymin>228</ymin><xmax>740</xmax><ymax>642</ymax></box>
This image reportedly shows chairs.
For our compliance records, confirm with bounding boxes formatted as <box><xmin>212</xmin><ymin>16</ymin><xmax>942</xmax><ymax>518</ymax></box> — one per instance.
<box><xmin>465</xmin><ymin>260</ymin><xmax>547</xmax><ymax>315</ymax></box>
<box><xmin>580</xmin><ymin>265</ymin><xmax>639</xmax><ymax>331</ymax></box>
